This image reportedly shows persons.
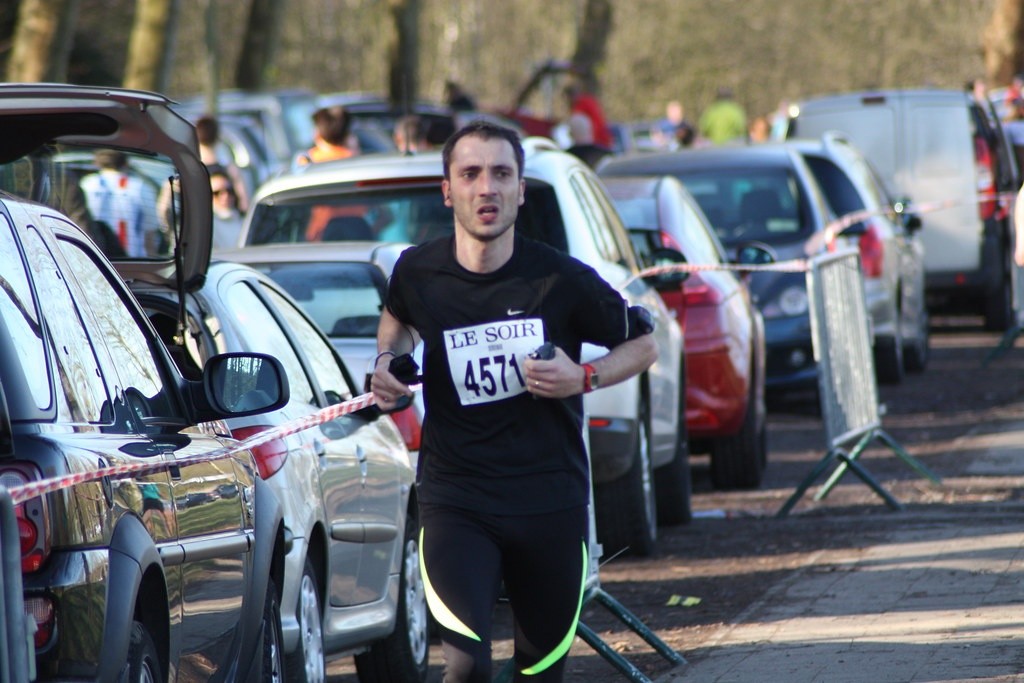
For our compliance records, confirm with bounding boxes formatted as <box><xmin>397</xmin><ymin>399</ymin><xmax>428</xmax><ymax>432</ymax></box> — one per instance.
<box><xmin>76</xmin><ymin>115</ymin><xmax>255</xmax><ymax>257</ymax></box>
<box><xmin>370</xmin><ymin>120</ymin><xmax>660</xmax><ymax>683</ymax></box>
<box><xmin>289</xmin><ymin>76</ymin><xmax>771</xmax><ymax>174</ymax></box>
<box><xmin>966</xmin><ymin>72</ymin><xmax>1024</xmax><ymax>122</ymax></box>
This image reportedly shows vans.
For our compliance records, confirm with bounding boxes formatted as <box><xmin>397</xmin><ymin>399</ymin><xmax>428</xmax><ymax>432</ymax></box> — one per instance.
<box><xmin>781</xmin><ymin>86</ymin><xmax>1024</xmax><ymax>388</ymax></box>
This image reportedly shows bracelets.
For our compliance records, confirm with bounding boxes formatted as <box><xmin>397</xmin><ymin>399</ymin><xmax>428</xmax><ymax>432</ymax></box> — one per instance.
<box><xmin>373</xmin><ymin>350</ymin><xmax>397</xmax><ymax>370</ymax></box>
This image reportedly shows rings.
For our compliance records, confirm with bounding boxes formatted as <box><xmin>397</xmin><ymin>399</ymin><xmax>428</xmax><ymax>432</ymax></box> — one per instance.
<box><xmin>534</xmin><ymin>379</ymin><xmax>540</xmax><ymax>388</ymax></box>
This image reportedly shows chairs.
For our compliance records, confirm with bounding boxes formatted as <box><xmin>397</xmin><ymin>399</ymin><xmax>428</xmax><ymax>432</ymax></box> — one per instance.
<box><xmin>739</xmin><ymin>189</ymin><xmax>786</xmax><ymax>221</ymax></box>
<box><xmin>322</xmin><ymin>215</ymin><xmax>378</xmax><ymax>241</ymax></box>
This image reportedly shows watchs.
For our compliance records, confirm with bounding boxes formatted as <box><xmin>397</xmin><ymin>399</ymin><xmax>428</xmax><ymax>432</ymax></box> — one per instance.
<box><xmin>582</xmin><ymin>363</ymin><xmax>600</xmax><ymax>393</ymax></box>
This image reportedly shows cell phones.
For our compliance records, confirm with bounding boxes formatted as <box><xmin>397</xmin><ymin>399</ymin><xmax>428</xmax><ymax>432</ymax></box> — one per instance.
<box><xmin>534</xmin><ymin>342</ymin><xmax>556</xmax><ymax>359</ymax></box>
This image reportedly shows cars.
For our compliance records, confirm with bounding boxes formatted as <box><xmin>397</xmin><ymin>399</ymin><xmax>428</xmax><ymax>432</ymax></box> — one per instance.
<box><xmin>0</xmin><ymin>79</ymin><xmax>427</xmax><ymax>682</ymax></box>
<box><xmin>165</xmin><ymin>92</ymin><xmax>929</xmax><ymax>560</ymax></box>
